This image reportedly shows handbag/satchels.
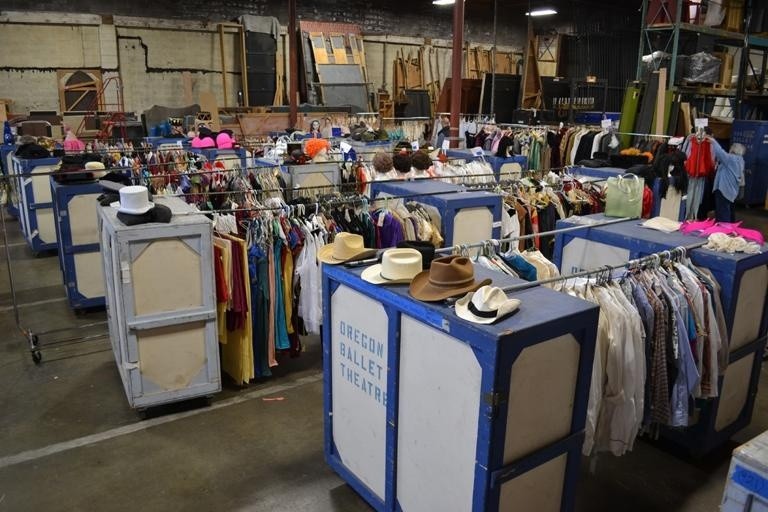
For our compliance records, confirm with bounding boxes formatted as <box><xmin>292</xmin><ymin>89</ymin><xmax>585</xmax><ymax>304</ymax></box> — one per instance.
<box><xmin>604</xmin><ymin>173</ymin><xmax>645</xmax><ymax>218</ymax></box>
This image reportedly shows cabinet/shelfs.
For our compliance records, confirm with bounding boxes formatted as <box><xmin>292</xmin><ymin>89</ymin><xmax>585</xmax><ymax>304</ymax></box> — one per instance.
<box><xmin>637</xmin><ymin>1</ymin><xmax>768</xmax><ymax>119</ymax></box>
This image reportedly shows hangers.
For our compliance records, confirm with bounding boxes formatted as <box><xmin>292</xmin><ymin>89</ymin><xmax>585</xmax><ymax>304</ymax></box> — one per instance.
<box><xmin>688</xmin><ymin>128</ymin><xmax>710</xmax><ymax>140</ymax></box>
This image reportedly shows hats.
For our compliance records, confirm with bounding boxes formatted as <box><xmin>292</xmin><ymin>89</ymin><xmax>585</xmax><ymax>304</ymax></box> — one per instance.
<box><xmin>192</xmin><ymin>137</ymin><xmax>200</xmax><ymax>148</ymax></box>
<box><xmin>216</xmin><ymin>132</ymin><xmax>233</xmax><ymax>150</ymax></box>
<box><xmin>360</xmin><ymin>248</ymin><xmax>423</xmax><ymax>285</ymax></box>
<box><xmin>455</xmin><ymin>284</ymin><xmax>521</xmax><ymax>325</ymax></box>
<box><xmin>200</xmin><ymin>137</ymin><xmax>215</xmax><ymax>148</ymax></box>
<box><xmin>305</xmin><ymin>138</ymin><xmax>330</xmax><ymax>158</ymax></box>
<box><xmin>96</xmin><ymin>172</ymin><xmax>133</xmax><ymax>192</ymax></box>
<box><xmin>410</xmin><ymin>255</ymin><xmax>492</xmax><ymax>302</ymax></box>
<box><xmin>316</xmin><ymin>232</ymin><xmax>379</xmax><ymax>265</ymax></box>
<box><xmin>380</xmin><ymin>241</ymin><xmax>443</xmax><ymax>270</ymax></box>
<box><xmin>85</xmin><ymin>162</ymin><xmax>108</xmax><ymax>178</ymax></box>
<box><xmin>110</xmin><ymin>185</ymin><xmax>156</xmax><ymax>215</ymax></box>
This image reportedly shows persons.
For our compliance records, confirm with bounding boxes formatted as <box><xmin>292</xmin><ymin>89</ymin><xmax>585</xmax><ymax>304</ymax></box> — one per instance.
<box><xmin>304</xmin><ymin>120</ymin><xmax>322</xmax><ymax>138</ymax></box>
<box><xmin>392</xmin><ymin>154</ymin><xmax>412</xmax><ymax>179</ymax></box>
<box><xmin>373</xmin><ymin>152</ymin><xmax>394</xmax><ymax>181</ymax></box>
<box><xmin>438</xmin><ymin>117</ymin><xmax>451</xmax><ymax>148</ymax></box>
<box><xmin>410</xmin><ymin>152</ymin><xmax>433</xmax><ymax>178</ymax></box>
<box><xmin>703</xmin><ymin>135</ymin><xmax>746</xmax><ymax>222</ymax></box>
<box><xmin>305</xmin><ymin>137</ymin><xmax>330</xmax><ymax>162</ymax></box>
